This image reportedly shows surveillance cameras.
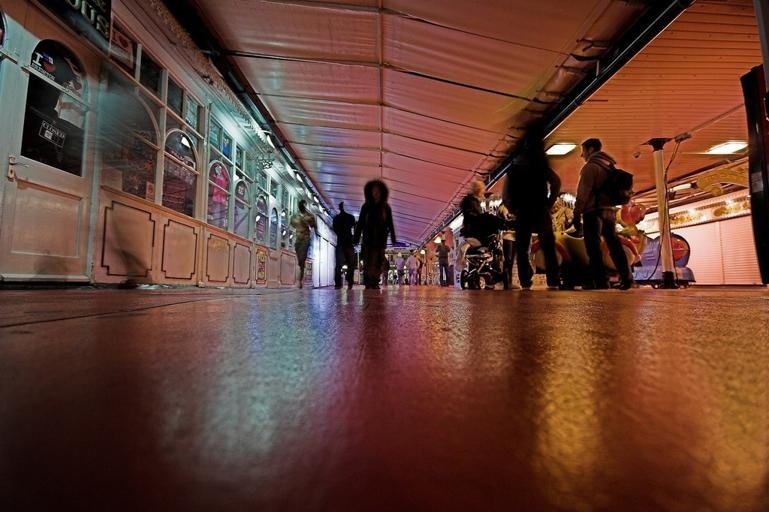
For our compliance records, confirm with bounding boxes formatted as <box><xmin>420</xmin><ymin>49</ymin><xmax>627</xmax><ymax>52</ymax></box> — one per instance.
<box><xmin>633</xmin><ymin>152</ymin><xmax>640</xmax><ymax>158</ymax></box>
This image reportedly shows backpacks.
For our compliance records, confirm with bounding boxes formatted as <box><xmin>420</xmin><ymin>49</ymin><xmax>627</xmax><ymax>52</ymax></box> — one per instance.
<box><xmin>588</xmin><ymin>157</ymin><xmax>634</xmax><ymax>206</ymax></box>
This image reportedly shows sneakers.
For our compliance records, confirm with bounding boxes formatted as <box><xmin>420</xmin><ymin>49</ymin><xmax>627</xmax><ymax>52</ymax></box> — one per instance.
<box><xmin>503</xmin><ymin>283</ymin><xmax>636</xmax><ymax>291</ymax></box>
<box><xmin>298</xmin><ymin>283</ymin><xmax>388</xmax><ymax>291</ymax></box>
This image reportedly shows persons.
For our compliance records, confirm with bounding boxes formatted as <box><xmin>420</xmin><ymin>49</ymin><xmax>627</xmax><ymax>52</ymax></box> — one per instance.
<box><xmin>383</xmin><ymin>238</ymin><xmax>459</xmax><ymax>285</ymax></box>
<box><xmin>458</xmin><ymin>177</ymin><xmax>537</xmax><ymax>291</ymax></box>
<box><xmin>289</xmin><ymin>198</ymin><xmax>317</xmax><ymax>287</ymax></box>
<box><xmin>330</xmin><ymin>199</ymin><xmax>355</xmax><ymax>290</ymax></box>
<box><xmin>572</xmin><ymin>137</ymin><xmax>635</xmax><ymax>290</ymax></box>
<box><xmin>351</xmin><ymin>179</ymin><xmax>400</xmax><ymax>290</ymax></box>
<box><xmin>501</xmin><ymin>147</ymin><xmax>561</xmax><ymax>291</ymax></box>
